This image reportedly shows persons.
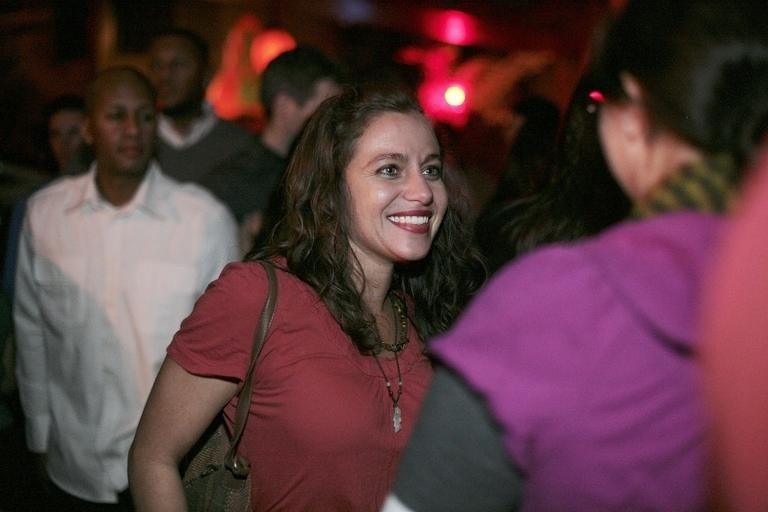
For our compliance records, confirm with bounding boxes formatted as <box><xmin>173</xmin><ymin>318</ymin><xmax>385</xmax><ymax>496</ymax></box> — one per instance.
<box><xmin>1</xmin><ymin>1</ymin><xmax>768</xmax><ymax>511</ymax></box>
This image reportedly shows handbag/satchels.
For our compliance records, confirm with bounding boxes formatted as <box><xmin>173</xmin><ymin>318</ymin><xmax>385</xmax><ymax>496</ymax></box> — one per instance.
<box><xmin>179</xmin><ymin>260</ymin><xmax>277</xmax><ymax>512</ymax></box>
